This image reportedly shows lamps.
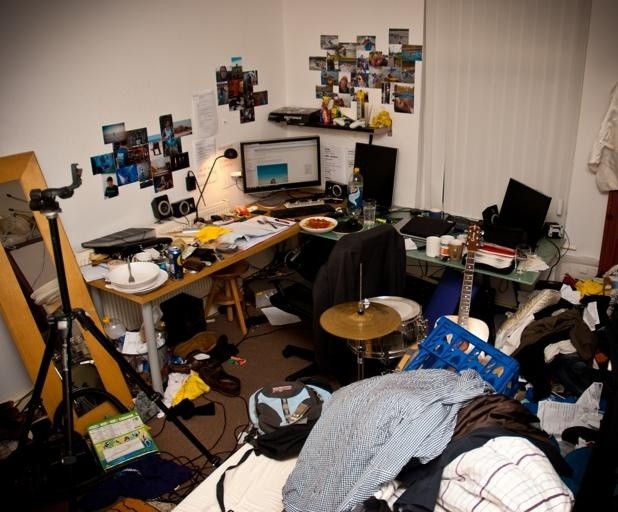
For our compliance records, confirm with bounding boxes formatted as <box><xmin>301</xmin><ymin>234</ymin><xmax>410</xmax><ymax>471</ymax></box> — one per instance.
<box><xmin>185</xmin><ymin>147</ymin><xmax>238</xmax><ymax>223</ymax></box>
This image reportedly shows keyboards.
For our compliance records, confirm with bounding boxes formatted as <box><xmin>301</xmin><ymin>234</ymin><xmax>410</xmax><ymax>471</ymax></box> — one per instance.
<box><xmin>272</xmin><ymin>204</ymin><xmax>335</xmax><ymax>218</ymax></box>
<box><xmin>462</xmin><ymin>251</ymin><xmax>520</xmax><ymax>274</ymax></box>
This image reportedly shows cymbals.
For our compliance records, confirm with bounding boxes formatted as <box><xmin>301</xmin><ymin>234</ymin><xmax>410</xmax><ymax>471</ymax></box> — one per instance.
<box><xmin>320</xmin><ymin>301</ymin><xmax>400</xmax><ymax>339</ymax></box>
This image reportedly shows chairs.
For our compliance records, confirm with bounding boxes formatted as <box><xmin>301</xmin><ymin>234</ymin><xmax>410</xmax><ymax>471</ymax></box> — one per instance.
<box><xmin>269</xmin><ymin>223</ymin><xmax>408</xmax><ymax>382</ymax></box>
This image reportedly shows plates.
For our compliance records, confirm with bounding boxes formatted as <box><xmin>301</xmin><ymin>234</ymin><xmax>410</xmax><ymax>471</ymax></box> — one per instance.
<box><xmin>332</xmin><ymin>220</ymin><xmax>363</xmax><ymax>235</ymax></box>
<box><xmin>108</xmin><ymin>262</ymin><xmax>167</xmax><ymax>296</ymax></box>
<box><xmin>298</xmin><ymin>215</ymin><xmax>337</xmax><ymax>234</ymax></box>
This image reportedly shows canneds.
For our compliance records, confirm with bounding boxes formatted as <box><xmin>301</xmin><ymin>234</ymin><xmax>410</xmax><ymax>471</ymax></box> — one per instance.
<box><xmin>168</xmin><ymin>246</ymin><xmax>183</xmax><ymax>281</ymax></box>
<box><xmin>440</xmin><ymin>235</ymin><xmax>455</xmax><ymax>262</ymax></box>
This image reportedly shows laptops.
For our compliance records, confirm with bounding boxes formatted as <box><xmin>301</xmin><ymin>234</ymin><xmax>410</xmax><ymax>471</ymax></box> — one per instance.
<box><xmin>400</xmin><ymin>216</ymin><xmax>456</xmax><ymax>238</ymax></box>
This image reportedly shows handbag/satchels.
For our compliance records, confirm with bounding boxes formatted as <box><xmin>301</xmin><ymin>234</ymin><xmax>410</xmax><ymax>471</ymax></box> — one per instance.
<box><xmin>245</xmin><ymin>424</ymin><xmax>312</xmax><ymax>460</ymax></box>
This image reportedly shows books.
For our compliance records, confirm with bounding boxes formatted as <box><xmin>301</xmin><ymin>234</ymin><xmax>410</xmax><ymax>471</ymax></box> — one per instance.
<box><xmin>212</xmin><ymin>214</ymin><xmax>296</xmax><ymax>252</ymax></box>
<box><xmin>81</xmin><ymin>227</ymin><xmax>156</xmax><ymax>247</ymax></box>
<box><xmin>87</xmin><ymin>412</ymin><xmax>160</xmax><ymax>474</ymax></box>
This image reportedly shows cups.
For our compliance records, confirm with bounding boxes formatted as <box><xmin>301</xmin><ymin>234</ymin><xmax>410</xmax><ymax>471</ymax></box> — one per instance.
<box><xmin>361</xmin><ymin>199</ymin><xmax>377</xmax><ymax>225</ymax></box>
<box><xmin>514</xmin><ymin>244</ymin><xmax>531</xmax><ymax>277</ymax></box>
<box><xmin>426</xmin><ymin>233</ymin><xmax>465</xmax><ymax>261</ymax></box>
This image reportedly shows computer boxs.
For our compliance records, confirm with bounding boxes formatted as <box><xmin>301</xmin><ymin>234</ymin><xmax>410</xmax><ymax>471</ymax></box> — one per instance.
<box><xmin>244</xmin><ymin>278</ymin><xmax>294</xmax><ymax>308</ymax></box>
<box><xmin>159</xmin><ymin>292</ymin><xmax>206</xmax><ymax>340</ymax></box>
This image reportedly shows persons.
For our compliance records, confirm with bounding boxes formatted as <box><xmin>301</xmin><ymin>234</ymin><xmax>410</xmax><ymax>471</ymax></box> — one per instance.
<box><xmin>368</xmin><ymin>52</ymin><xmax>387</xmax><ymax>67</ymax></box>
<box><xmin>361</xmin><ymin>35</ymin><xmax>375</xmax><ymax>50</ymax></box>
<box><xmin>339</xmin><ymin>75</ymin><xmax>349</xmax><ymax>93</ymax></box>
<box><xmin>356</xmin><ymin>73</ymin><xmax>366</xmax><ymax>86</ymax></box>
<box><xmin>104</xmin><ymin>176</ymin><xmax>118</xmax><ymax>199</ymax></box>
<box><xmin>161</xmin><ymin>120</ymin><xmax>173</xmax><ymax>138</ymax></box>
<box><xmin>219</xmin><ymin>66</ymin><xmax>227</xmax><ymax>80</ymax></box>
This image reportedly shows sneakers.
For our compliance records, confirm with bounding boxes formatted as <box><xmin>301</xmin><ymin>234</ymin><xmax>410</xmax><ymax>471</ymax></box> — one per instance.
<box><xmin>200</xmin><ymin>360</ymin><xmax>242</xmax><ymax>397</ymax></box>
<box><xmin>172</xmin><ymin>330</ymin><xmax>218</xmax><ymax>361</ymax></box>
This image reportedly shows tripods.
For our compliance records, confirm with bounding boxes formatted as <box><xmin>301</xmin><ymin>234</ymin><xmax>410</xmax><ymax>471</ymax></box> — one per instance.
<box><xmin>15</xmin><ymin>309</ymin><xmax>219</xmax><ymax>512</ymax></box>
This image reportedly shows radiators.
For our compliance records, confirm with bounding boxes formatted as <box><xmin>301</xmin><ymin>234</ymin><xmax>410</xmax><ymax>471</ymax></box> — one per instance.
<box><xmin>95</xmin><ymin>198</ymin><xmax>232</xmax><ymax>331</ymax></box>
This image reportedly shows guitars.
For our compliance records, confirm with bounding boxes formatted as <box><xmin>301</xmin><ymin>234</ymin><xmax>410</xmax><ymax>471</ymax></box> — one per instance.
<box><xmin>434</xmin><ymin>224</ymin><xmax>489</xmax><ymax>357</ymax></box>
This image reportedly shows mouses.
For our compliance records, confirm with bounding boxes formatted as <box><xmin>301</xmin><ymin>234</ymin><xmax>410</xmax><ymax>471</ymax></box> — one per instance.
<box><xmin>349</xmin><ymin>218</ymin><xmax>358</xmax><ymax>227</ymax></box>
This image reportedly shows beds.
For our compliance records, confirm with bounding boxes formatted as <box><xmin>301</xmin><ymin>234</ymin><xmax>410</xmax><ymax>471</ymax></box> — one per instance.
<box><xmin>170</xmin><ymin>443</ymin><xmax>307</xmax><ymax>512</ymax></box>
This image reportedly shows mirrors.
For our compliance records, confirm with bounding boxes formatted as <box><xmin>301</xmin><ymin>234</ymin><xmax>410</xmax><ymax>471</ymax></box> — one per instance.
<box><xmin>0</xmin><ymin>150</ymin><xmax>135</xmax><ymax>436</ymax></box>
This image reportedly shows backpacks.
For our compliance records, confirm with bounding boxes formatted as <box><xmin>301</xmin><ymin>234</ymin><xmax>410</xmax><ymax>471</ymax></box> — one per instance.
<box><xmin>248</xmin><ymin>380</ymin><xmax>332</xmax><ymax>438</ymax></box>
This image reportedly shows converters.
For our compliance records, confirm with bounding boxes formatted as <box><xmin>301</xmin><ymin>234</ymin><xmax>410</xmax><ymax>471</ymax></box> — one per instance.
<box><xmin>186</xmin><ymin>175</ymin><xmax>195</xmax><ymax>191</ymax></box>
<box><xmin>546</xmin><ymin>222</ymin><xmax>563</xmax><ymax>239</ymax></box>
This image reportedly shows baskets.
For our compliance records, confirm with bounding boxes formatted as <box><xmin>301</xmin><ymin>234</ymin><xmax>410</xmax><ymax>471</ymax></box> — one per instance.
<box><xmin>402</xmin><ymin>315</ymin><xmax>520</xmax><ymax>399</ymax></box>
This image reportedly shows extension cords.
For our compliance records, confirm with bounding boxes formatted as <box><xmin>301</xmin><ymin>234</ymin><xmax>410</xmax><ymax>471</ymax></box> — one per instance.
<box><xmin>284</xmin><ymin>199</ymin><xmax>326</xmax><ymax>209</ymax></box>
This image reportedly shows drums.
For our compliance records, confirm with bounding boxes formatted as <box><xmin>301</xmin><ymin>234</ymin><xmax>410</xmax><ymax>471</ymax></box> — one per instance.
<box><xmin>345</xmin><ymin>296</ymin><xmax>424</xmax><ymax>360</ymax></box>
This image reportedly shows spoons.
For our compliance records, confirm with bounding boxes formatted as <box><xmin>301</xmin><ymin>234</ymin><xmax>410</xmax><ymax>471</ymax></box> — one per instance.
<box><xmin>126</xmin><ymin>255</ymin><xmax>134</xmax><ymax>283</ymax></box>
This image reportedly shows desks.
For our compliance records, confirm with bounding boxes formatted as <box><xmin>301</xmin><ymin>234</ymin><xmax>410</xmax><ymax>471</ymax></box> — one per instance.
<box><xmin>302</xmin><ymin>200</ymin><xmax>568</xmax><ymax>308</ymax></box>
<box><xmin>74</xmin><ymin>179</ymin><xmax>347</xmax><ymax>401</ymax></box>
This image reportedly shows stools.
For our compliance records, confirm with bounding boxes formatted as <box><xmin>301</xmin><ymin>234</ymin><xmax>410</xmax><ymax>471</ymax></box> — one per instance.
<box><xmin>203</xmin><ymin>260</ymin><xmax>252</xmax><ymax>336</ymax></box>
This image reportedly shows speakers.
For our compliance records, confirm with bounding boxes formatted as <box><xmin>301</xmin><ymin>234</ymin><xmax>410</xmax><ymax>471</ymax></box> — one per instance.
<box><xmin>152</xmin><ymin>195</ymin><xmax>172</xmax><ymax>223</ymax></box>
<box><xmin>326</xmin><ymin>180</ymin><xmax>347</xmax><ymax>199</ymax></box>
<box><xmin>172</xmin><ymin>197</ymin><xmax>197</xmax><ymax>218</ymax></box>
<box><xmin>482</xmin><ymin>205</ymin><xmax>499</xmax><ymax>237</ymax></box>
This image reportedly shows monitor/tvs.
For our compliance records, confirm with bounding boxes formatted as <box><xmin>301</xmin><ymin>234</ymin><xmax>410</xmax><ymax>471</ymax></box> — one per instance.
<box><xmin>490</xmin><ymin>177</ymin><xmax>552</xmax><ymax>254</ymax></box>
<box><xmin>240</xmin><ymin>136</ymin><xmax>321</xmax><ymax>206</ymax></box>
<box><xmin>355</xmin><ymin>142</ymin><xmax>397</xmax><ymax>208</ymax></box>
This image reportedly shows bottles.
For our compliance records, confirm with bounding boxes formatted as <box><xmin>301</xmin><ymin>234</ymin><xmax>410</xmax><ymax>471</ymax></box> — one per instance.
<box><xmin>345</xmin><ymin>167</ymin><xmax>363</xmax><ymax>220</ymax></box>
<box><xmin>102</xmin><ymin>316</ymin><xmax>127</xmax><ymax>345</ymax></box>
